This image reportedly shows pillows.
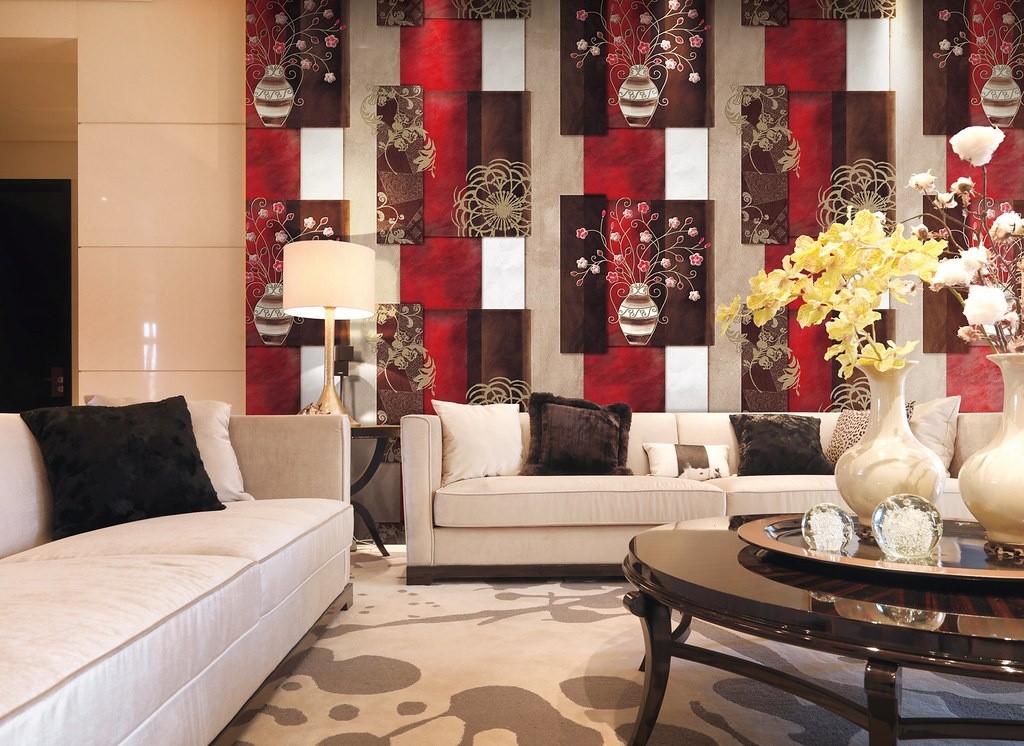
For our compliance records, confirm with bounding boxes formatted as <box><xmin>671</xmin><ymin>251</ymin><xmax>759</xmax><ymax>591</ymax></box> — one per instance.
<box><xmin>909</xmin><ymin>395</ymin><xmax>962</xmax><ymax>478</ymax></box>
<box><xmin>20</xmin><ymin>395</ymin><xmax>226</xmax><ymax>541</ymax></box>
<box><xmin>518</xmin><ymin>393</ymin><xmax>634</xmax><ymax>477</ymax></box>
<box><xmin>826</xmin><ymin>400</ymin><xmax>916</xmax><ymax>468</ymax></box>
<box><xmin>431</xmin><ymin>399</ymin><xmax>523</xmax><ymax>488</ymax></box>
<box><xmin>729</xmin><ymin>414</ymin><xmax>834</xmax><ymax>477</ymax></box>
<box><xmin>642</xmin><ymin>443</ymin><xmax>731</xmax><ymax>482</ymax></box>
<box><xmin>83</xmin><ymin>394</ymin><xmax>256</xmax><ymax>502</ymax></box>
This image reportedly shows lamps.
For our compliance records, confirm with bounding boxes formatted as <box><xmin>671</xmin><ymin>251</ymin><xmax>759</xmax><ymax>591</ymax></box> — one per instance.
<box><xmin>282</xmin><ymin>240</ymin><xmax>376</xmax><ymax>427</ymax></box>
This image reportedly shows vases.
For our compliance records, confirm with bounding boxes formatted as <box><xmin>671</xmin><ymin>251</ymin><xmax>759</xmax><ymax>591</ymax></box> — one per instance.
<box><xmin>834</xmin><ymin>360</ymin><xmax>947</xmax><ymax>526</ymax></box>
<box><xmin>958</xmin><ymin>353</ymin><xmax>1024</xmax><ymax>544</ymax></box>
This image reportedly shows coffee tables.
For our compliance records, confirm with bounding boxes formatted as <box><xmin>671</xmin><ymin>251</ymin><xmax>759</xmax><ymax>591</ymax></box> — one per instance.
<box><xmin>621</xmin><ymin>514</ymin><xmax>1024</xmax><ymax>746</ymax></box>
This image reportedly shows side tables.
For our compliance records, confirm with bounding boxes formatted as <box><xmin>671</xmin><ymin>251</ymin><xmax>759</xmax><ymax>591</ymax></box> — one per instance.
<box><xmin>351</xmin><ymin>424</ymin><xmax>401</xmax><ymax>557</ymax></box>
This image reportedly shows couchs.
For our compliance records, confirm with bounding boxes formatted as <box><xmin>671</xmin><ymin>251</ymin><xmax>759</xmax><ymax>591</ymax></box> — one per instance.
<box><xmin>0</xmin><ymin>413</ymin><xmax>353</xmax><ymax>746</ymax></box>
<box><xmin>400</xmin><ymin>411</ymin><xmax>1003</xmax><ymax>586</ymax></box>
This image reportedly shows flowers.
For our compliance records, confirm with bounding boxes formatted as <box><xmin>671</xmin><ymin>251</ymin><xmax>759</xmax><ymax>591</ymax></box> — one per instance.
<box><xmin>904</xmin><ymin>125</ymin><xmax>1024</xmax><ymax>353</ymax></box>
<box><xmin>715</xmin><ymin>209</ymin><xmax>949</xmax><ymax>380</ymax></box>
